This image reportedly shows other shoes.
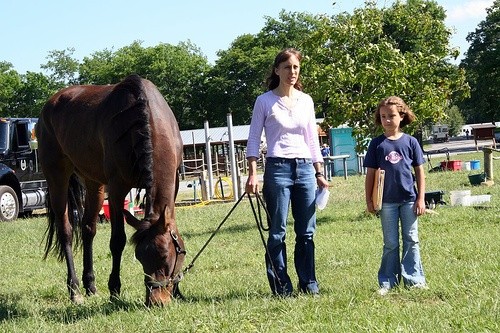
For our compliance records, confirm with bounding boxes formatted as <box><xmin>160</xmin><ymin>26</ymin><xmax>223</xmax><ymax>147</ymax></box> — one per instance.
<box><xmin>409</xmin><ymin>284</ymin><xmax>430</xmax><ymax>292</ymax></box>
<box><xmin>375</xmin><ymin>281</ymin><xmax>391</xmax><ymax>297</ymax></box>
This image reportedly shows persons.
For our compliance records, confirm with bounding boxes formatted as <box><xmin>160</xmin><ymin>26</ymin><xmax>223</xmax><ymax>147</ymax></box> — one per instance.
<box><xmin>321</xmin><ymin>142</ymin><xmax>329</xmax><ymax>157</ymax></box>
<box><xmin>361</xmin><ymin>96</ymin><xmax>431</xmax><ymax>296</ymax></box>
<box><xmin>245</xmin><ymin>48</ymin><xmax>329</xmax><ymax>300</ymax></box>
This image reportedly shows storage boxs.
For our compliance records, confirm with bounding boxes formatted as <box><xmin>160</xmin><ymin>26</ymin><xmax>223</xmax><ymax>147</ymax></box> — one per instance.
<box><xmin>440</xmin><ymin>160</ymin><xmax>462</xmax><ymax>171</ymax></box>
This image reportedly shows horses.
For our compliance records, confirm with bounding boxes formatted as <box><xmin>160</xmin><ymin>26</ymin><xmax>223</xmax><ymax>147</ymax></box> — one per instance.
<box><xmin>33</xmin><ymin>74</ymin><xmax>187</xmax><ymax>308</ymax></box>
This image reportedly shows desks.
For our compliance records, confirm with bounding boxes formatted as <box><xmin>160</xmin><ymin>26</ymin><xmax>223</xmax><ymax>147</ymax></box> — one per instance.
<box><xmin>323</xmin><ymin>155</ymin><xmax>350</xmax><ymax>181</ymax></box>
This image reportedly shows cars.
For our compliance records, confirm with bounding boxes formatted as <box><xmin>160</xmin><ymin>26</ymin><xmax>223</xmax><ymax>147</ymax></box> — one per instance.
<box><xmin>0</xmin><ymin>118</ymin><xmax>48</xmax><ymax>222</ymax></box>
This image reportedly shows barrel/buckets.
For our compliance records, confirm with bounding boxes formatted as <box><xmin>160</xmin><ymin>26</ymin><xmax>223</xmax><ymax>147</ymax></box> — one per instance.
<box><xmin>463</xmin><ymin>162</ymin><xmax>470</xmax><ymax>170</ymax></box>
<box><xmin>101</xmin><ymin>199</ymin><xmax>130</xmax><ymax>223</ymax></box>
<box><xmin>470</xmin><ymin>160</ymin><xmax>480</xmax><ymax>170</ymax></box>
<box><xmin>451</xmin><ymin>189</ymin><xmax>471</xmax><ymax>206</ymax></box>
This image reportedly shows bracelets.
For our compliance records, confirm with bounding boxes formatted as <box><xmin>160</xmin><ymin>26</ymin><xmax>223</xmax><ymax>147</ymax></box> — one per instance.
<box><xmin>316</xmin><ymin>171</ymin><xmax>324</xmax><ymax>177</ymax></box>
<box><xmin>365</xmin><ymin>199</ymin><xmax>373</xmax><ymax>206</ymax></box>
